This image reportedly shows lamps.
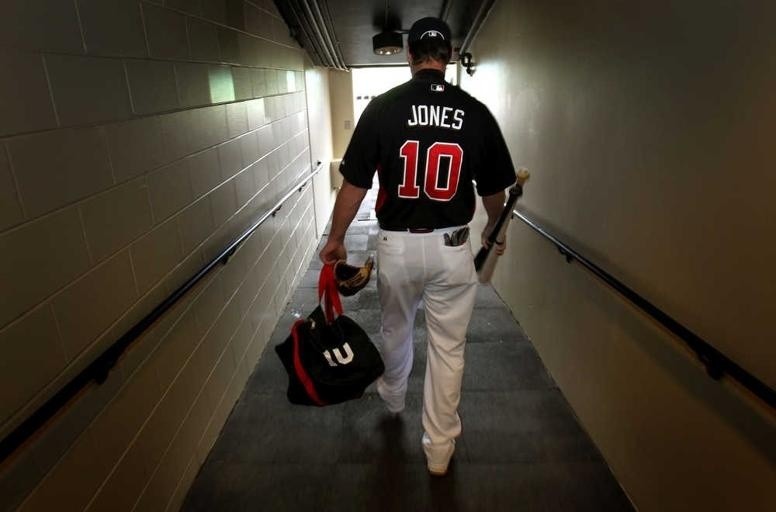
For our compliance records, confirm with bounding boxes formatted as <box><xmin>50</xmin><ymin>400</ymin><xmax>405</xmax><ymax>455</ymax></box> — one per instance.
<box><xmin>370</xmin><ymin>29</ymin><xmax>403</xmax><ymax>57</ymax></box>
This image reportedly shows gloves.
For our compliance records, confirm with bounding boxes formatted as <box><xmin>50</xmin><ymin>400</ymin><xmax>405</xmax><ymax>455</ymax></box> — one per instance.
<box><xmin>444</xmin><ymin>227</ymin><xmax>469</xmax><ymax>246</ymax></box>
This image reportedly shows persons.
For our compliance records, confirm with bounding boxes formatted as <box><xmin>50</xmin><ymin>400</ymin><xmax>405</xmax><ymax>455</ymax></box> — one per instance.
<box><xmin>318</xmin><ymin>16</ymin><xmax>517</xmax><ymax>478</ymax></box>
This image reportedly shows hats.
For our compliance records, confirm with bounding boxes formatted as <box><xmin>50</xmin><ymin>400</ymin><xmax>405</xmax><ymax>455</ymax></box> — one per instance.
<box><xmin>408</xmin><ymin>17</ymin><xmax>451</xmax><ymax>44</ymax></box>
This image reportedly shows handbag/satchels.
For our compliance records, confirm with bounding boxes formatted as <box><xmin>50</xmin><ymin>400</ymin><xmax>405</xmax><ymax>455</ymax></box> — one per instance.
<box><xmin>275</xmin><ymin>304</ymin><xmax>385</xmax><ymax>407</ymax></box>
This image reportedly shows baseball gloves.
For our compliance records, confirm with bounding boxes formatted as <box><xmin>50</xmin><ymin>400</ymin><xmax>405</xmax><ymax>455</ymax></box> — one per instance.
<box><xmin>332</xmin><ymin>254</ymin><xmax>374</xmax><ymax>296</ymax></box>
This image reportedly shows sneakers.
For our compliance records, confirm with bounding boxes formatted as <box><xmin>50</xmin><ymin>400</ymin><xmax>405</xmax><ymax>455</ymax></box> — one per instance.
<box><xmin>427</xmin><ymin>458</ymin><xmax>448</xmax><ymax>475</ymax></box>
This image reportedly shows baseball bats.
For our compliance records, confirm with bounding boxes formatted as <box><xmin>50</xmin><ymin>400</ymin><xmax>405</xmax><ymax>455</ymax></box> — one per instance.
<box><xmin>474</xmin><ymin>167</ymin><xmax>530</xmax><ymax>284</ymax></box>
